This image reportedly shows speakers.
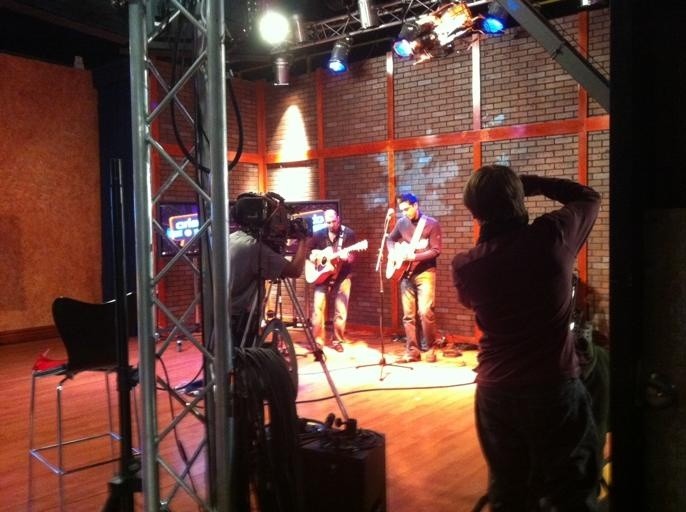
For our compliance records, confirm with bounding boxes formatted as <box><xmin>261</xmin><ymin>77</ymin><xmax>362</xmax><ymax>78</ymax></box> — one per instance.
<box><xmin>250</xmin><ymin>428</ymin><xmax>386</xmax><ymax>512</ymax></box>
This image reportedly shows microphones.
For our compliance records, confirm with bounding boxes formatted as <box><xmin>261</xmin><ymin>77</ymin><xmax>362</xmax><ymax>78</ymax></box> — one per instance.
<box><xmin>385</xmin><ymin>208</ymin><xmax>394</xmax><ymax>220</ymax></box>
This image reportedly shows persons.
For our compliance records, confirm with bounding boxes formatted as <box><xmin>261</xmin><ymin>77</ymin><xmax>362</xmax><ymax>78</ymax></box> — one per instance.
<box><xmin>227</xmin><ymin>192</ymin><xmax>315</xmax><ymax>511</ymax></box>
<box><xmin>386</xmin><ymin>193</ymin><xmax>442</xmax><ymax>363</ymax></box>
<box><xmin>303</xmin><ymin>209</ymin><xmax>357</xmax><ymax>354</ymax></box>
<box><xmin>451</xmin><ymin>164</ymin><xmax>602</xmax><ymax>512</ymax></box>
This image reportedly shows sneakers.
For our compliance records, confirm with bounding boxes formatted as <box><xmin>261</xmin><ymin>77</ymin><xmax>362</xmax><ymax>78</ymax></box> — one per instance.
<box><xmin>335</xmin><ymin>343</ymin><xmax>343</xmax><ymax>352</ymax></box>
<box><xmin>426</xmin><ymin>350</ymin><xmax>435</xmax><ymax>362</ymax></box>
<box><xmin>397</xmin><ymin>353</ymin><xmax>421</xmax><ymax>364</ymax></box>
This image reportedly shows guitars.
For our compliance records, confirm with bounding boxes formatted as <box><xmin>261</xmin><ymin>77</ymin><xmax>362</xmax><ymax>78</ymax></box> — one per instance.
<box><xmin>303</xmin><ymin>239</ymin><xmax>369</xmax><ymax>285</ymax></box>
<box><xmin>386</xmin><ymin>239</ymin><xmax>429</xmax><ymax>282</ymax></box>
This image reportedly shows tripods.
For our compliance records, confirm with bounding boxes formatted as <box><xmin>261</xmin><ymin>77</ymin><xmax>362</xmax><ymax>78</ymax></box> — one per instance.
<box><xmin>356</xmin><ymin>217</ymin><xmax>413</xmax><ymax>381</ymax></box>
<box><xmin>279</xmin><ymin>282</ymin><xmax>307</xmax><ymax>358</ymax></box>
<box><xmin>262</xmin><ymin>277</ymin><xmax>349</xmax><ymax>421</ymax></box>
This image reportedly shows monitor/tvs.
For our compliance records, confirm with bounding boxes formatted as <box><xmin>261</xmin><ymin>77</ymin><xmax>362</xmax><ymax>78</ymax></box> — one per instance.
<box><xmin>285</xmin><ymin>199</ymin><xmax>340</xmax><ymax>263</ymax></box>
<box><xmin>156</xmin><ymin>201</ymin><xmax>201</xmax><ymax>257</ymax></box>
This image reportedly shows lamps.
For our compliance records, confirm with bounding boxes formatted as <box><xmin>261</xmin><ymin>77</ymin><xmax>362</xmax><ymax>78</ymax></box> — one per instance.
<box><xmin>273</xmin><ymin>1</ymin><xmax>517</xmax><ymax>97</ymax></box>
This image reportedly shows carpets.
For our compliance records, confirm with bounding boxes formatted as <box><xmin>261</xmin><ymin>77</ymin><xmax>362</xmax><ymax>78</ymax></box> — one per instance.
<box><xmin>278</xmin><ymin>332</ymin><xmax>480</xmax><ymax>404</ymax></box>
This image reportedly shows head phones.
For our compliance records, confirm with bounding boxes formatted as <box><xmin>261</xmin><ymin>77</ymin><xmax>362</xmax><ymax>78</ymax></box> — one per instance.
<box><xmin>231</xmin><ymin>192</ymin><xmax>255</xmax><ymax>216</ymax></box>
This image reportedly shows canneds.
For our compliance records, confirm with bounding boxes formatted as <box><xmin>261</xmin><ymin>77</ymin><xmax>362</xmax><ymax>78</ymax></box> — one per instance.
<box><xmin>176</xmin><ymin>339</ymin><xmax>183</xmax><ymax>352</ymax></box>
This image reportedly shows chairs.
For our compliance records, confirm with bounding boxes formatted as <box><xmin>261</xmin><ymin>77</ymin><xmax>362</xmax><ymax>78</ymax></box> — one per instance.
<box><xmin>26</xmin><ymin>290</ymin><xmax>145</xmax><ymax>479</ymax></box>
